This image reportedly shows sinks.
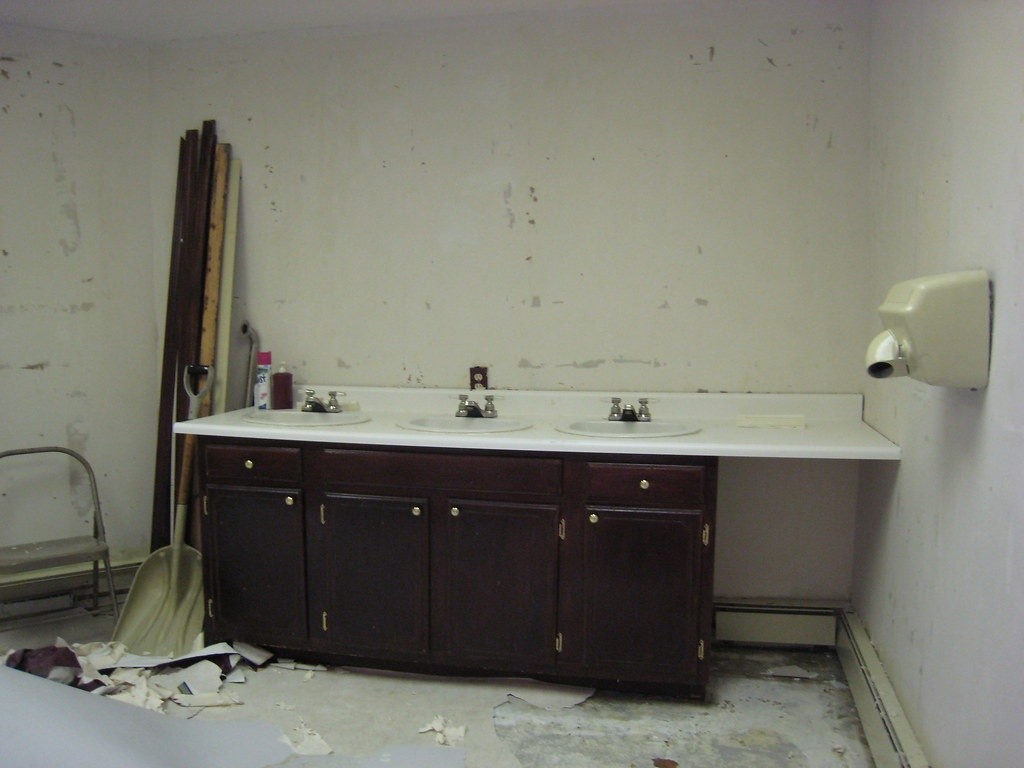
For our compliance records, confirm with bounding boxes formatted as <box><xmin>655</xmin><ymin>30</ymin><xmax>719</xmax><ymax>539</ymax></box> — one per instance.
<box><xmin>242</xmin><ymin>406</ymin><xmax>372</xmax><ymax>427</ymax></box>
<box><xmin>551</xmin><ymin>415</ymin><xmax>702</xmax><ymax>439</ymax></box>
<box><xmin>395</xmin><ymin>411</ymin><xmax>533</xmax><ymax>434</ymax></box>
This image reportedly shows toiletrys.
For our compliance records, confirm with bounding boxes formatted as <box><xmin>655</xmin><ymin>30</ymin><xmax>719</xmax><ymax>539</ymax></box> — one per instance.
<box><xmin>252</xmin><ymin>349</ymin><xmax>272</xmax><ymax>410</ymax></box>
<box><xmin>272</xmin><ymin>359</ymin><xmax>293</xmax><ymax>408</ymax></box>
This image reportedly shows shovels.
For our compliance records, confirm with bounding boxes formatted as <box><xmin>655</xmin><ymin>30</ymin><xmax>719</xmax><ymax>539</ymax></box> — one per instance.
<box><xmin>107</xmin><ymin>364</ymin><xmax>215</xmax><ymax>656</ymax></box>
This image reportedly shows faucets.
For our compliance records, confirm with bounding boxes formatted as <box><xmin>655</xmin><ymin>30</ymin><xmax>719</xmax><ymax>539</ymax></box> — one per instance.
<box><xmin>609</xmin><ymin>396</ymin><xmax>649</xmax><ymax>421</ymax></box>
<box><xmin>301</xmin><ymin>388</ymin><xmax>346</xmax><ymax>412</ymax></box>
<box><xmin>456</xmin><ymin>394</ymin><xmax>499</xmax><ymax>416</ymax></box>
